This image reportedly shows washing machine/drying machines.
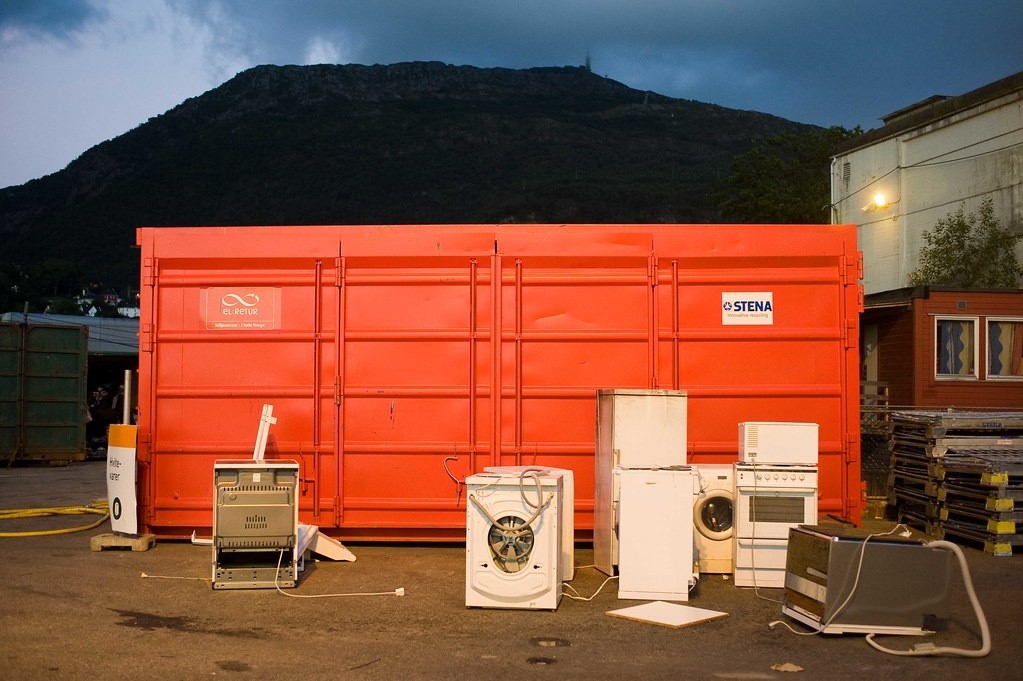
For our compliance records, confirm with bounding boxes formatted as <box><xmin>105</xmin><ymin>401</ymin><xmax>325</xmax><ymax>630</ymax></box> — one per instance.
<box><xmin>684</xmin><ymin>456</ymin><xmax>748</xmax><ymax>582</ymax></box>
<box><xmin>457</xmin><ymin>462</ymin><xmax>576</xmax><ymax>619</ymax></box>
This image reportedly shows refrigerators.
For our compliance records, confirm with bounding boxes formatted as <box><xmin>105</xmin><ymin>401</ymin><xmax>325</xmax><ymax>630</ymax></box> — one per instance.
<box><xmin>612</xmin><ymin>469</ymin><xmax>698</xmax><ymax>602</ymax></box>
<box><xmin>593</xmin><ymin>389</ymin><xmax>688</xmax><ymax>576</ymax></box>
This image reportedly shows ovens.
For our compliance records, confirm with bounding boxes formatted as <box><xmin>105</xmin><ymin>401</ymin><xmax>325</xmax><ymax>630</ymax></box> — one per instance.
<box><xmin>731</xmin><ymin>462</ymin><xmax>819</xmax><ymax>589</ymax></box>
